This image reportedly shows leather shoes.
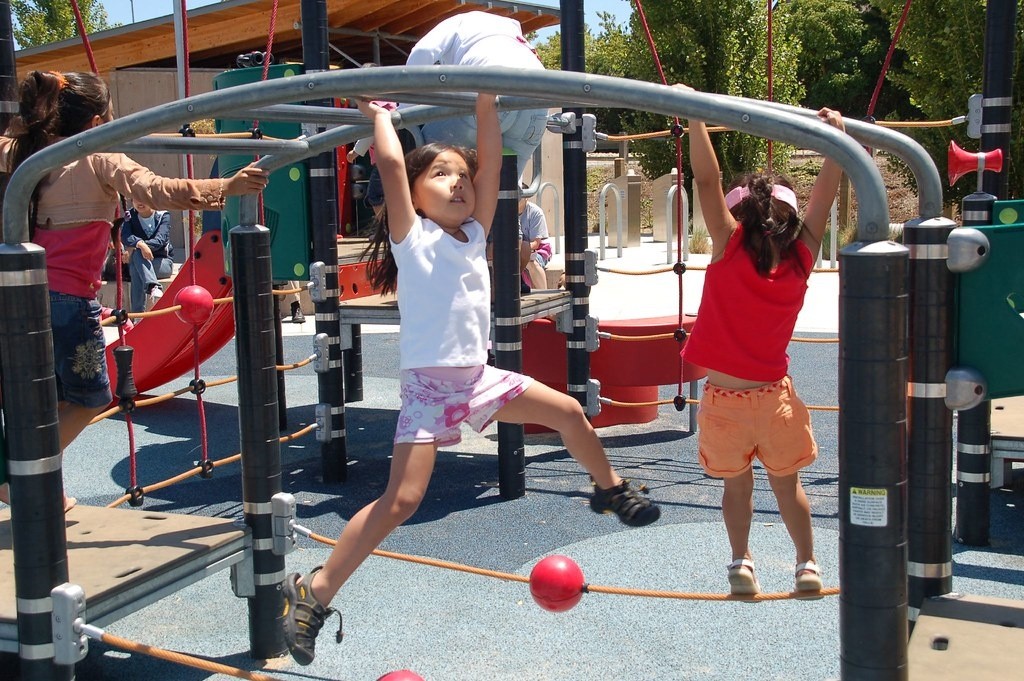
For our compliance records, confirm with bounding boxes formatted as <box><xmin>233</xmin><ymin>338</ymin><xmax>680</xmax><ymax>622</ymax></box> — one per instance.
<box><xmin>291</xmin><ymin>308</ymin><xmax>305</xmax><ymax>322</ymax></box>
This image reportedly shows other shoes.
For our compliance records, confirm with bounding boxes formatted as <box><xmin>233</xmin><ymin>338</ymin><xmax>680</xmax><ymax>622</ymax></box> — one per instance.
<box><xmin>147</xmin><ymin>285</ymin><xmax>163</xmax><ymax>310</ymax></box>
<box><xmin>64</xmin><ymin>496</ymin><xmax>77</xmax><ymax>513</ymax></box>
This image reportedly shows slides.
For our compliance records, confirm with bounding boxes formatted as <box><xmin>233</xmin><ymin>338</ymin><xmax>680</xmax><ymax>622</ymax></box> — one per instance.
<box><xmin>106</xmin><ymin>229</ymin><xmax>236</xmax><ymax>406</ymax></box>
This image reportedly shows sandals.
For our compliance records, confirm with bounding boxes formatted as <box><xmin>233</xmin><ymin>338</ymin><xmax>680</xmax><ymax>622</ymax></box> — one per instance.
<box><xmin>793</xmin><ymin>563</ymin><xmax>824</xmax><ymax>601</ymax></box>
<box><xmin>589</xmin><ymin>476</ymin><xmax>660</xmax><ymax>526</ymax></box>
<box><xmin>727</xmin><ymin>560</ymin><xmax>761</xmax><ymax>603</ymax></box>
<box><xmin>281</xmin><ymin>566</ymin><xmax>344</xmax><ymax>666</ymax></box>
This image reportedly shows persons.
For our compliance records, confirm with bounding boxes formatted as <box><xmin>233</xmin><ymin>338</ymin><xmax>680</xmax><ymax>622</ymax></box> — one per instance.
<box><xmin>281</xmin><ymin>92</ymin><xmax>661</xmax><ymax>667</ymax></box>
<box><xmin>346</xmin><ymin>63</ymin><xmax>385</xmax><ymax>222</ymax></box>
<box><xmin>0</xmin><ymin>71</ymin><xmax>269</xmax><ymax>513</ymax></box>
<box><xmin>519</xmin><ymin>182</ymin><xmax>552</xmax><ymax>290</ymax></box>
<box><xmin>120</xmin><ymin>199</ymin><xmax>173</xmax><ymax>325</ymax></box>
<box><xmin>672</xmin><ymin>84</ymin><xmax>846</xmax><ymax>595</ymax></box>
<box><xmin>406</xmin><ymin>11</ymin><xmax>550</xmax><ymax>182</ymax></box>
